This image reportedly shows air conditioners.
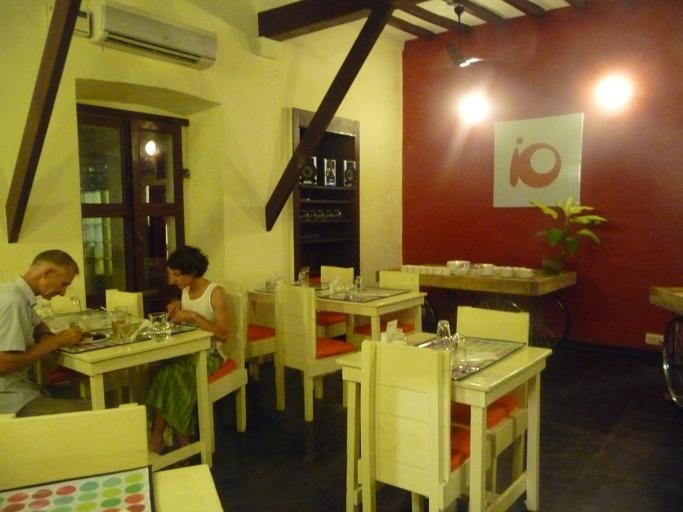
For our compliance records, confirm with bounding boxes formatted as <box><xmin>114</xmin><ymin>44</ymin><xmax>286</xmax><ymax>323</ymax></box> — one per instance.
<box><xmin>84</xmin><ymin>2</ymin><xmax>219</xmax><ymax>72</ymax></box>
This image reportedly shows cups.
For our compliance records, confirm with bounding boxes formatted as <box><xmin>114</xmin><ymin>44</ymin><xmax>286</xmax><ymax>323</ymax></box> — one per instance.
<box><xmin>109</xmin><ymin>304</ymin><xmax>130</xmax><ymax>345</ymax></box>
<box><xmin>146</xmin><ymin>311</ymin><xmax>171</xmax><ymax>344</ymax></box>
<box><xmin>298</xmin><ymin>207</ymin><xmax>343</xmax><ymax>219</ymax></box>
<box><xmin>297</xmin><ymin>271</ymin><xmax>368</xmax><ymax>300</ymax></box>
<box><xmin>450</xmin><ymin>333</ymin><xmax>468</xmax><ymax>370</ymax></box>
<box><xmin>400</xmin><ymin>264</ymin><xmax>451</xmax><ymax>277</ymax></box>
<box><xmin>435</xmin><ymin>320</ymin><xmax>452</xmax><ymax>349</ymax></box>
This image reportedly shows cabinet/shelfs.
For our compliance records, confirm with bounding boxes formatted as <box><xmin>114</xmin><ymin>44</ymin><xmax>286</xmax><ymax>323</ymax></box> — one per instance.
<box><xmin>288</xmin><ymin>104</ymin><xmax>361</xmax><ymax>283</ymax></box>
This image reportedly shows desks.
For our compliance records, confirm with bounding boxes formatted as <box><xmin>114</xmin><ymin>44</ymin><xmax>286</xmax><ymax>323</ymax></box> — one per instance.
<box><xmin>648</xmin><ymin>283</ymin><xmax>682</xmax><ymax>415</ymax></box>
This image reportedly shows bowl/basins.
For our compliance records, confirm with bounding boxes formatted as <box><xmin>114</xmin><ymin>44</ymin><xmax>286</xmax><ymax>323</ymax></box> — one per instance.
<box><xmin>446</xmin><ymin>260</ymin><xmax>535</xmax><ymax>281</ymax></box>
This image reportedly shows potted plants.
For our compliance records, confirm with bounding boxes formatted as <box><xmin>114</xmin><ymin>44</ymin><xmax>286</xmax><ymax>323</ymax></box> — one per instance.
<box><xmin>527</xmin><ymin>194</ymin><xmax>604</xmax><ymax>277</ymax></box>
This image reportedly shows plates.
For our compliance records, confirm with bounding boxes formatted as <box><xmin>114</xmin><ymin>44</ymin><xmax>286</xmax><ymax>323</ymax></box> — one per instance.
<box><xmin>145</xmin><ymin>321</ymin><xmax>174</xmax><ymax>331</ymax></box>
<box><xmin>76</xmin><ymin>331</ymin><xmax>110</xmax><ymax>345</ymax></box>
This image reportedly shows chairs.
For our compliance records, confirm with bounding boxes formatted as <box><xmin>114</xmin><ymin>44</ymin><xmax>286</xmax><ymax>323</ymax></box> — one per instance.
<box><xmin>0</xmin><ymin>264</ymin><xmax>530</xmax><ymax>511</ymax></box>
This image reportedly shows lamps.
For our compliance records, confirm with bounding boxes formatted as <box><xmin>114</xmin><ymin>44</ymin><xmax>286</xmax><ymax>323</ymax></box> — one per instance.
<box><xmin>444</xmin><ymin>1</ymin><xmax>479</xmax><ymax>70</ymax></box>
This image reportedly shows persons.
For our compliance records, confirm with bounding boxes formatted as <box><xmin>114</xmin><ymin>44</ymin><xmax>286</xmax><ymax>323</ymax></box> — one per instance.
<box><xmin>0</xmin><ymin>249</ymin><xmax>93</xmax><ymax>416</ymax></box>
<box><xmin>138</xmin><ymin>246</ymin><xmax>230</xmax><ymax>471</ymax></box>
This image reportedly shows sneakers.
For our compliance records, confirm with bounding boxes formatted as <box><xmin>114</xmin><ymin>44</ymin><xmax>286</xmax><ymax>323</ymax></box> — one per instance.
<box><xmin>148</xmin><ymin>435</ymin><xmax>168</xmax><ymax>453</ymax></box>
